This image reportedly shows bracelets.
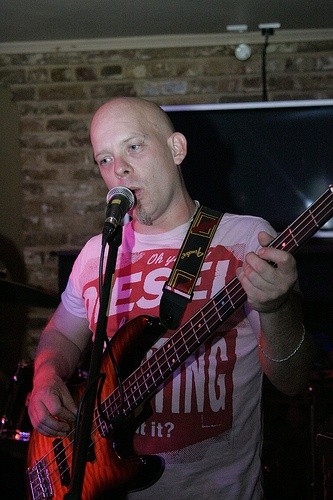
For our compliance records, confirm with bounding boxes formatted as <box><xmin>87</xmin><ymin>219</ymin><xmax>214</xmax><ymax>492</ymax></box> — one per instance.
<box><xmin>257</xmin><ymin>320</ymin><xmax>306</xmax><ymax>364</ymax></box>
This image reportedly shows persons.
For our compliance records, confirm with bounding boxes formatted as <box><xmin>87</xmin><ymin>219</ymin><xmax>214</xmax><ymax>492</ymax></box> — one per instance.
<box><xmin>26</xmin><ymin>96</ymin><xmax>313</xmax><ymax>500</ymax></box>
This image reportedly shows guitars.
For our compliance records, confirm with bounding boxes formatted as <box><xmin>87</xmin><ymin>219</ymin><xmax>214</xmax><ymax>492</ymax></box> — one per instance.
<box><xmin>24</xmin><ymin>180</ymin><xmax>333</xmax><ymax>500</ymax></box>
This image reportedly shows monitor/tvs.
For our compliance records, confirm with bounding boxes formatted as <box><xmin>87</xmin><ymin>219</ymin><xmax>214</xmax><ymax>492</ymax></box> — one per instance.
<box><xmin>158</xmin><ymin>98</ymin><xmax>333</xmax><ymax>237</ymax></box>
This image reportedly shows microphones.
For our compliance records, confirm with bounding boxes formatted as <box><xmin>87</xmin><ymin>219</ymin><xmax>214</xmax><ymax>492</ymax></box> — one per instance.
<box><xmin>103</xmin><ymin>186</ymin><xmax>135</xmax><ymax>242</ymax></box>
